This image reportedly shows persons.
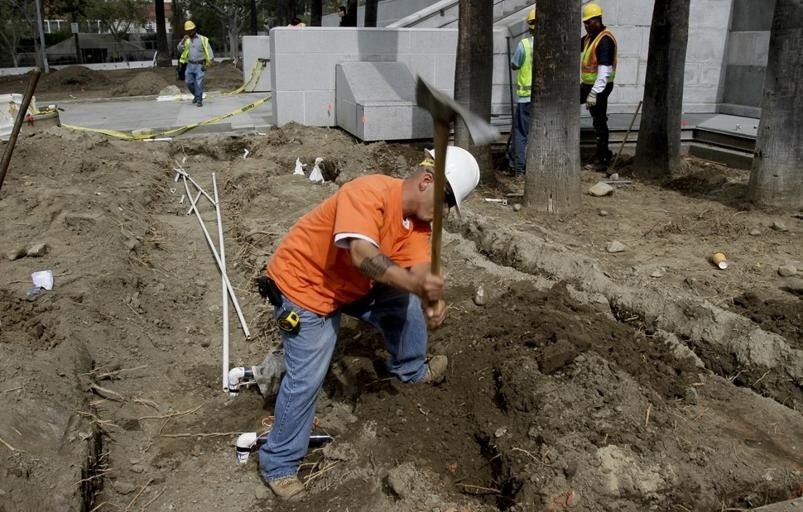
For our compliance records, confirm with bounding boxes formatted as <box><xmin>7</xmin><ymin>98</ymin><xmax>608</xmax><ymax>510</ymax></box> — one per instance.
<box><xmin>579</xmin><ymin>1</ymin><xmax>619</xmax><ymax>172</ymax></box>
<box><xmin>175</xmin><ymin>19</ymin><xmax>215</xmax><ymax>107</ymax></box>
<box><xmin>336</xmin><ymin>6</ymin><xmax>348</xmax><ymax>27</ymax></box>
<box><xmin>510</xmin><ymin>8</ymin><xmax>535</xmax><ymax>174</ymax></box>
<box><xmin>256</xmin><ymin>144</ymin><xmax>480</xmax><ymax>500</ymax></box>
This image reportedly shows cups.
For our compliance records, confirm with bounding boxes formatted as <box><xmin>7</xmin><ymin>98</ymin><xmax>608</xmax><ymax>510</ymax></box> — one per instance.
<box><xmin>711</xmin><ymin>252</ymin><xmax>728</xmax><ymax>270</ymax></box>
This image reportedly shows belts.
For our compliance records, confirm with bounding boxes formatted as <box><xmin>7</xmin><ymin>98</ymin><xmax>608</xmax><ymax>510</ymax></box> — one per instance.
<box><xmin>189</xmin><ymin>61</ymin><xmax>203</xmax><ymax>64</ymax></box>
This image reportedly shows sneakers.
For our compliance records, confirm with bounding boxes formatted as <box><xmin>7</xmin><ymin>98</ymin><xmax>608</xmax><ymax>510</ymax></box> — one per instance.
<box><xmin>501</xmin><ymin>168</ymin><xmax>523</xmax><ymax>179</ymax></box>
<box><xmin>267</xmin><ymin>475</ymin><xmax>307</xmax><ymax>502</ymax></box>
<box><xmin>424</xmin><ymin>353</ymin><xmax>448</xmax><ymax>385</ymax></box>
<box><xmin>193</xmin><ymin>97</ymin><xmax>203</xmax><ymax>107</ymax></box>
<box><xmin>582</xmin><ymin>154</ymin><xmax>611</xmax><ymax>170</ymax></box>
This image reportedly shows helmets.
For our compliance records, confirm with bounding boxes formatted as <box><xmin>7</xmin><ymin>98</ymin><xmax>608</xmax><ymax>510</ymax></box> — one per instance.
<box><xmin>527</xmin><ymin>9</ymin><xmax>535</xmax><ymax>21</ymax></box>
<box><xmin>423</xmin><ymin>145</ymin><xmax>480</xmax><ymax>219</ymax></box>
<box><xmin>581</xmin><ymin>3</ymin><xmax>603</xmax><ymax>22</ymax></box>
<box><xmin>184</xmin><ymin>21</ymin><xmax>196</xmax><ymax>31</ymax></box>
